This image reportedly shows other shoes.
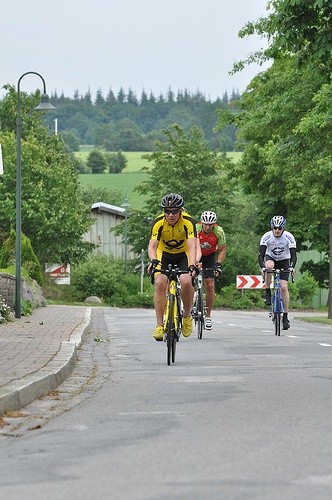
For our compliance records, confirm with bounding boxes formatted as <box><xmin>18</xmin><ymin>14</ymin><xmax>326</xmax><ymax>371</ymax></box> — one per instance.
<box><xmin>265</xmin><ymin>292</ymin><xmax>272</xmax><ymax>305</ymax></box>
<box><xmin>282</xmin><ymin>318</ymin><xmax>290</xmax><ymax>329</ymax></box>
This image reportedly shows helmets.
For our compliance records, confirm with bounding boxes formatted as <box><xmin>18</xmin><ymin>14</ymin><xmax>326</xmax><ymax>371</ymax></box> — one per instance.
<box><xmin>200</xmin><ymin>211</ymin><xmax>218</xmax><ymax>224</ymax></box>
<box><xmin>270</xmin><ymin>216</ymin><xmax>287</xmax><ymax>230</ymax></box>
<box><xmin>160</xmin><ymin>193</ymin><xmax>185</xmax><ymax>209</ymax></box>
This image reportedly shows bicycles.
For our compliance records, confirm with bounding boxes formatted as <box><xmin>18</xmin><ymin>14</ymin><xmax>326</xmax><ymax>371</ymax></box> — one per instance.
<box><xmin>188</xmin><ymin>265</ymin><xmax>221</xmax><ymax>340</ymax></box>
<box><xmin>262</xmin><ymin>267</ymin><xmax>295</xmax><ymax>335</ymax></box>
<box><xmin>146</xmin><ymin>257</ymin><xmax>204</xmax><ymax>366</ymax></box>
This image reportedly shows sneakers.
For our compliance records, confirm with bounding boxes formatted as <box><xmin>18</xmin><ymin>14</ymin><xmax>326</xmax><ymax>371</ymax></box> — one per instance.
<box><xmin>152</xmin><ymin>326</ymin><xmax>164</xmax><ymax>339</ymax></box>
<box><xmin>181</xmin><ymin>315</ymin><xmax>194</xmax><ymax>337</ymax></box>
<box><xmin>204</xmin><ymin>316</ymin><xmax>214</xmax><ymax>331</ymax></box>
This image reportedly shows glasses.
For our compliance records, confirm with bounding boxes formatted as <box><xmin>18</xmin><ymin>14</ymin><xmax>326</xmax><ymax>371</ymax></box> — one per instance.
<box><xmin>273</xmin><ymin>226</ymin><xmax>283</xmax><ymax>230</ymax></box>
<box><xmin>163</xmin><ymin>210</ymin><xmax>180</xmax><ymax>214</ymax></box>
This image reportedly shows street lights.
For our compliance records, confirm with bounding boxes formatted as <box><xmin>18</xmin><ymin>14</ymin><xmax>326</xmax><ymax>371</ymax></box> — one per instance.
<box><xmin>15</xmin><ymin>71</ymin><xmax>58</xmax><ymax>317</ymax></box>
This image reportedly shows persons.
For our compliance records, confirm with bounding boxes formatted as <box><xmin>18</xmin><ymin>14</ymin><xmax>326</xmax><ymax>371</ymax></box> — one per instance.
<box><xmin>195</xmin><ymin>211</ymin><xmax>226</xmax><ymax>331</ymax></box>
<box><xmin>258</xmin><ymin>216</ymin><xmax>298</xmax><ymax>329</ymax></box>
<box><xmin>147</xmin><ymin>193</ymin><xmax>197</xmax><ymax>341</ymax></box>
